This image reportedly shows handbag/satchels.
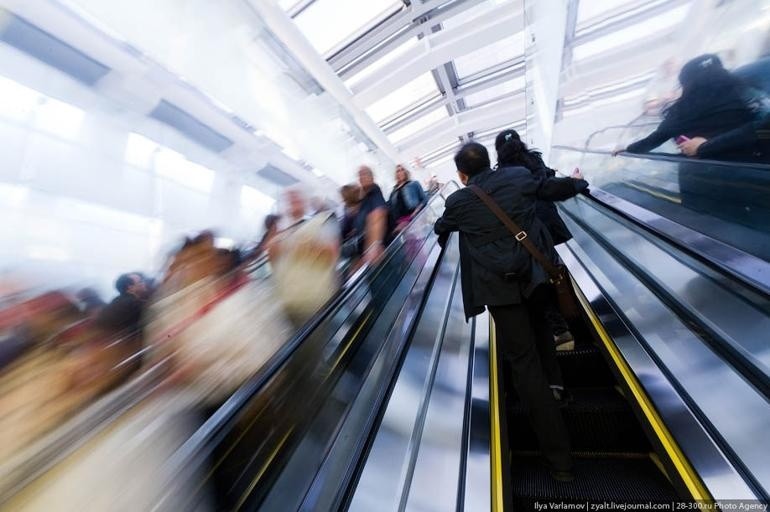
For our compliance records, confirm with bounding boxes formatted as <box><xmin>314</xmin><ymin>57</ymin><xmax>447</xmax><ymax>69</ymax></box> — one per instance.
<box><xmin>549</xmin><ymin>263</ymin><xmax>577</xmax><ymax>310</ymax></box>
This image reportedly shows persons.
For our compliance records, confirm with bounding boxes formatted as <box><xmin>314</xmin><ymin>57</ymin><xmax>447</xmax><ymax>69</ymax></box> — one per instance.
<box><xmin>3</xmin><ymin>159</ymin><xmax>445</xmax><ymax>511</ymax></box>
<box><xmin>611</xmin><ymin>52</ymin><xmax>770</xmax><ymax>212</ymax></box>
<box><xmin>434</xmin><ymin>142</ymin><xmax>591</xmax><ymax>486</ymax></box>
<box><xmin>490</xmin><ymin>130</ymin><xmax>583</xmax><ymax>410</ymax></box>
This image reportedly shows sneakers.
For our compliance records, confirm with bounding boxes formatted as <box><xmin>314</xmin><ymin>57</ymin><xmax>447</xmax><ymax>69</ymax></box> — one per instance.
<box><xmin>554</xmin><ymin>330</ymin><xmax>576</xmax><ymax>353</ymax></box>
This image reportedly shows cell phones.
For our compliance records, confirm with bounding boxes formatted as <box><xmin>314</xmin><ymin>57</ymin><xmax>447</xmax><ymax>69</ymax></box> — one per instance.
<box><xmin>676</xmin><ymin>134</ymin><xmax>688</xmax><ymax>144</ymax></box>
